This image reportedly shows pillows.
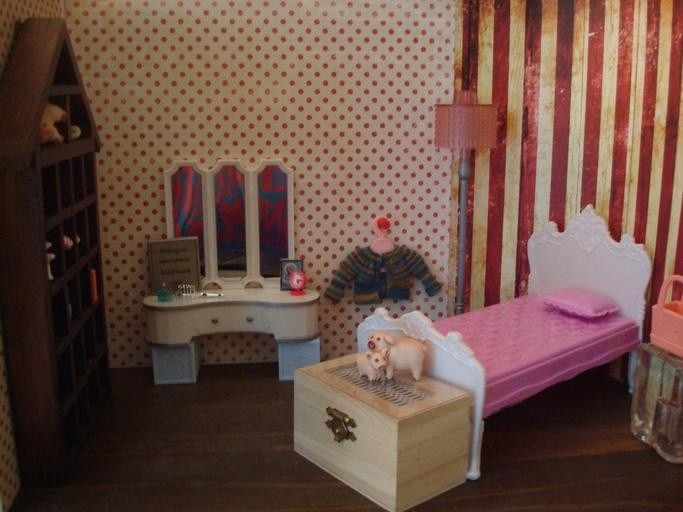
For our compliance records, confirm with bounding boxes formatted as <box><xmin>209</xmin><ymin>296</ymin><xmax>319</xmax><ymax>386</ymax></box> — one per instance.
<box><xmin>541</xmin><ymin>286</ymin><xmax>621</xmax><ymax>321</ymax></box>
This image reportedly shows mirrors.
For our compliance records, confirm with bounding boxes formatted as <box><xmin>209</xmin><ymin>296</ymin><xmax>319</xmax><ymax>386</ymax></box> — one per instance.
<box><xmin>210</xmin><ymin>160</ymin><xmax>252</xmax><ymax>291</ymax></box>
<box><xmin>162</xmin><ymin>159</ymin><xmax>210</xmax><ymax>290</ymax></box>
<box><xmin>252</xmin><ymin>159</ymin><xmax>295</xmax><ymax>288</ymax></box>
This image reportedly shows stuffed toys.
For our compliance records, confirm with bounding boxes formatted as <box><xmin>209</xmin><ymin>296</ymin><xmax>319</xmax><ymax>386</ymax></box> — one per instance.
<box><xmin>367</xmin><ymin>331</ymin><xmax>427</xmax><ymax>382</ymax></box>
<box><xmin>39</xmin><ymin>102</ymin><xmax>67</xmax><ymax>146</ymax></box>
<box><xmin>70</xmin><ymin>125</ymin><xmax>84</xmax><ymax>142</ymax></box>
<box><xmin>355</xmin><ymin>346</ymin><xmax>390</xmax><ymax>384</ymax></box>
<box><xmin>44</xmin><ymin>232</ymin><xmax>99</xmax><ymax>325</ymax></box>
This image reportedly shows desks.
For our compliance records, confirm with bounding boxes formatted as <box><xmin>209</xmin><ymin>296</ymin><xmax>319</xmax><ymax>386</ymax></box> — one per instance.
<box><xmin>140</xmin><ymin>288</ymin><xmax>322</xmax><ymax>385</ymax></box>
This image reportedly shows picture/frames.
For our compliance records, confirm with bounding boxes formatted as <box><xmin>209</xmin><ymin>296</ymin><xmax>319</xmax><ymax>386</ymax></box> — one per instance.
<box><xmin>146</xmin><ymin>236</ymin><xmax>200</xmax><ymax>294</ymax></box>
<box><xmin>280</xmin><ymin>257</ymin><xmax>303</xmax><ymax>290</ymax></box>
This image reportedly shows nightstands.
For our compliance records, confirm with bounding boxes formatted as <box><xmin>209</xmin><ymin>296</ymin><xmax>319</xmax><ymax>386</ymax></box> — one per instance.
<box><xmin>629</xmin><ymin>342</ymin><xmax>682</xmax><ymax>464</ymax></box>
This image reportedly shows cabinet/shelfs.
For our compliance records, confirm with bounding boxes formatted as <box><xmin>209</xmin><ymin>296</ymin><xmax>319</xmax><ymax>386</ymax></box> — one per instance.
<box><xmin>1</xmin><ymin>16</ymin><xmax>112</xmax><ymax>485</ymax></box>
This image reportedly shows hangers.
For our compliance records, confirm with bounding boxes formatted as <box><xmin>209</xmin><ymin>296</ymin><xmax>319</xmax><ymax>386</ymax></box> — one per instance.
<box><xmin>370</xmin><ymin>217</ymin><xmax>397</xmax><ymax>273</ymax></box>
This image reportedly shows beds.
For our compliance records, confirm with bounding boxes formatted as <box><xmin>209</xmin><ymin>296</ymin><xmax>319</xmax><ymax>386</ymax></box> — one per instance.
<box><xmin>356</xmin><ymin>202</ymin><xmax>653</xmax><ymax>481</ymax></box>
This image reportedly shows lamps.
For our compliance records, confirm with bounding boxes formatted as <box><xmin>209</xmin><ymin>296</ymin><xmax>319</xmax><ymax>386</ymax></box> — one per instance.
<box><xmin>434</xmin><ymin>90</ymin><xmax>499</xmax><ymax>315</ymax></box>
